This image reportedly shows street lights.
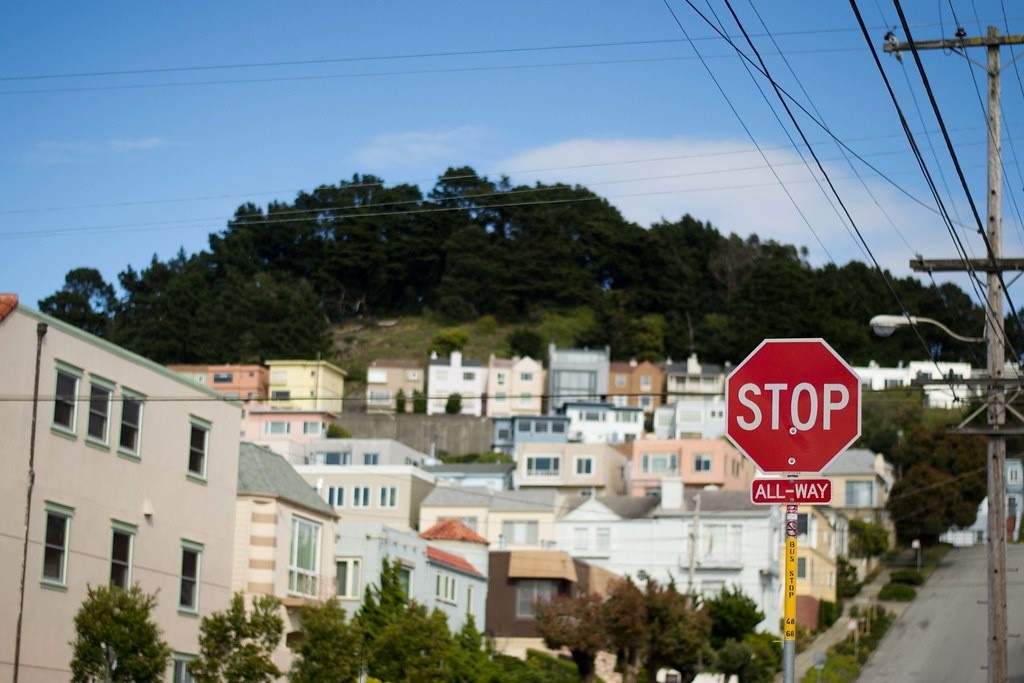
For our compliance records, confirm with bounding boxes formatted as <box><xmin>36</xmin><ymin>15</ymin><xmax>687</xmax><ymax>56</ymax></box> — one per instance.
<box><xmin>868</xmin><ymin>314</ymin><xmax>1010</xmax><ymax>683</ymax></box>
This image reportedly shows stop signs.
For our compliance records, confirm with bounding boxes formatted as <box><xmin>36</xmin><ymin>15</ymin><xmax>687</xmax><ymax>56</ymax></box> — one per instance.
<box><xmin>721</xmin><ymin>339</ymin><xmax>862</xmax><ymax>476</ymax></box>
